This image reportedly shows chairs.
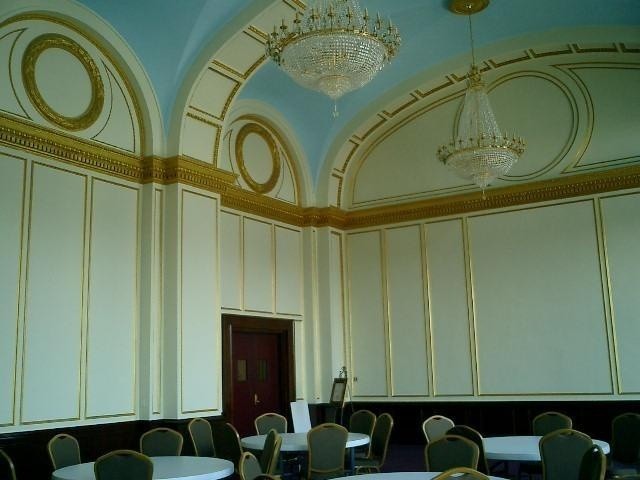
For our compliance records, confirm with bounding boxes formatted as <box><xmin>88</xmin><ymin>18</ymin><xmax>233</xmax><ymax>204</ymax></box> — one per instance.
<box><xmin>138</xmin><ymin>427</ymin><xmax>183</xmax><ymax>456</ymax></box>
<box><xmin>260</xmin><ymin>429</ymin><xmax>275</xmax><ymax>472</ymax></box>
<box><xmin>306</xmin><ymin>423</ymin><xmax>349</xmax><ymax>479</ymax></box>
<box><xmin>432</xmin><ymin>467</ymin><xmax>488</xmax><ymax>480</ymax></box>
<box><xmin>93</xmin><ymin>450</ymin><xmax>154</xmax><ymax>479</ymax></box>
<box><xmin>578</xmin><ymin>444</ymin><xmax>606</xmax><ymax>477</ymax></box>
<box><xmin>532</xmin><ymin>412</ymin><xmax>573</xmax><ymax>434</ymax></box>
<box><xmin>1</xmin><ymin>449</ymin><xmax>16</xmax><ymax>479</ymax></box>
<box><xmin>422</xmin><ymin>415</ymin><xmax>454</xmax><ymax>442</ymax></box>
<box><xmin>189</xmin><ymin>417</ymin><xmax>216</xmax><ymax>456</ymax></box>
<box><xmin>424</xmin><ymin>435</ymin><xmax>480</xmax><ymax>470</ymax></box>
<box><xmin>225</xmin><ymin>423</ymin><xmax>242</xmax><ymax>462</ymax></box>
<box><xmin>257</xmin><ymin>473</ymin><xmax>282</xmax><ymax>478</ymax></box>
<box><xmin>47</xmin><ymin>433</ymin><xmax>83</xmax><ymax>469</ymax></box>
<box><xmin>444</xmin><ymin>424</ymin><xmax>488</xmax><ymax>473</ymax></box>
<box><xmin>354</xmin><ymin>414</ymin><xmax>393</xmax><ymax>473</ymax></box>
<box><xmin>349</xmin><ymin>411</ymin><xmax>375</xmax><ymax>453</ymax></box>
<box><xmin>239</xmin><ymin>452</ymin><xmax>262</xmax><ymax>477</ymax></box>
<box><xmin>254</xmin><ymin>412</ymin><xmax>287</xmax><ymax>435</ymax></box>
<box><xmin>538</xmin><ymin>428</ymin><xmax>594</xmax><ymax>478</ymax></box>
<box><xmin>264</xmin><ymin>437</ymin><xmax>283</xmax><ymax>473</ymax></box>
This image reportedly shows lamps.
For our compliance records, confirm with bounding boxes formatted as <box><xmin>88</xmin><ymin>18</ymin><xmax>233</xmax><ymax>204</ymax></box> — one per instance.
<box><xmin>435</xmin><ymin>4</ymin><xmax>526</xmax><ymax>201</ymax></box>
<box><xmin>263</xmin><ymin>0</ymin><xmax>402</xmax><ymax>122</ymax></box>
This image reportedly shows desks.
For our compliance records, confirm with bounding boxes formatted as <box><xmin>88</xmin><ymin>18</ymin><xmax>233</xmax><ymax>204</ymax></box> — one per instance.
<box><xmin>333</xmin><ymin>472</ymin><xmax>506</xmax><ymax>480</ymax></box>
<box><xmin>242</xmin><ymin>432</ymin><xmax>371</xmax><ymax>479</ymax></box>
<box><xmin>52</xmin><ymin>457</ymin><xmax>234</xmax><ymax>480</ymax></box>
<box><xmin>483</xmin><ymin>435</ymin><xmax>611</xmax><ymax>476</ymax></box>
